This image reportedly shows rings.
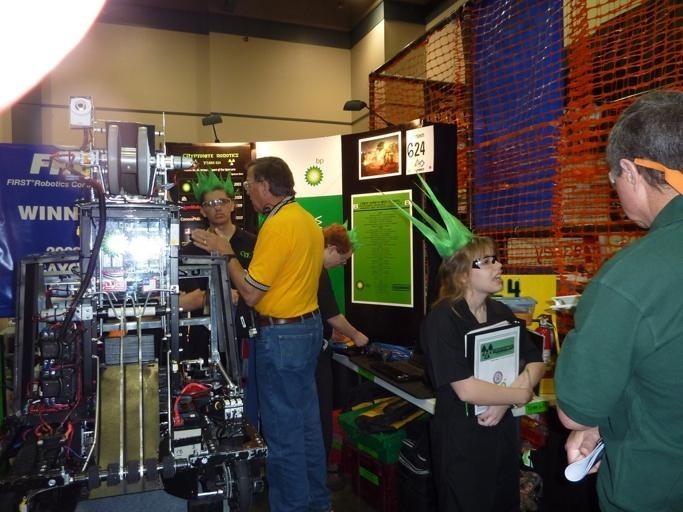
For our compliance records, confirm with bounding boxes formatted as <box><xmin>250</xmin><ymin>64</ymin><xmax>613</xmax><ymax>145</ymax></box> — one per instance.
<box><xmin>203</xmin><ymin>240</ymin><xmax>207</xmax><ymax>245</ymax></box>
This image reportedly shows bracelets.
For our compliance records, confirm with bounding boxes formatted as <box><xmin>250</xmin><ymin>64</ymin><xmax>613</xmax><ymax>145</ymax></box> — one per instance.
<box><xmin>224</xmin><ymin>254</ymin><xmax>237</xmax><ymax>264</ymax></box>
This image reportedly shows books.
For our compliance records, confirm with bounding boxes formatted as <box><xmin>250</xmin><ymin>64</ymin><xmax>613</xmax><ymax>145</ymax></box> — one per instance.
<box><xmin>462</xmin><ymin>320</ymin><xmax>521</xmax><ymax>418</ymax></box>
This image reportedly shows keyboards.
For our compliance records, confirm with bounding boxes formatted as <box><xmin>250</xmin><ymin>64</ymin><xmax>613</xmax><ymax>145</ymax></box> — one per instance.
<box><xmin>385</xmin><ymin>359</ymin><xmax>424</xmax><ymax>380</ymax></box>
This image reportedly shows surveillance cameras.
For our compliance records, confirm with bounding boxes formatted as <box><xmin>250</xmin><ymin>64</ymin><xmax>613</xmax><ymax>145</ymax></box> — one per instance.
<box><xmin>70</xmin><ymin>96</ymin><xmax>94</xmax><ymax>130</ymax></box>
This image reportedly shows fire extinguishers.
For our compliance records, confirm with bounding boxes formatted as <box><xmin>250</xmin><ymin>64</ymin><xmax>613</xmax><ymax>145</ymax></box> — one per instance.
<box><xmin>531</xmin><ymin>315</ymin><xmax>551</xmax><ymax>367</ymax></box>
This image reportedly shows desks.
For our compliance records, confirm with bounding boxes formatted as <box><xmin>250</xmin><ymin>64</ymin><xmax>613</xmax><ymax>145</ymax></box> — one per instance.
<box><xmin>332</xmin><ymin>347</ymin><xmax>546</xmax><ymax>417</ymax></box>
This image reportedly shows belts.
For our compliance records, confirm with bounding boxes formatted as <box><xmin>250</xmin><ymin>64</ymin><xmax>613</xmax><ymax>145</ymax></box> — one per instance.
<box><xmin>259</xmin><ymin>309</ymin><xmax>319</xmax><ymax>325</ymax></box>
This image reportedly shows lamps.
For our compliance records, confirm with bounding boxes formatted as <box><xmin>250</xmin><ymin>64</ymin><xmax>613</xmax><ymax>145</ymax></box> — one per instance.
<box><xmin>202</xmin><ymin>115</ymin><xmax>223</xmax><ymax>143</ymax></box>
<box><xmin>344</xmin><ymin>100</ymin><xmax>392</xmax><ymax>126</ymax></box>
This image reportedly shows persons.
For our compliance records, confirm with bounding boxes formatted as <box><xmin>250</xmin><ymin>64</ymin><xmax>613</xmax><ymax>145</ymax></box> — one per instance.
<box><xmin>189</xmin><ymin>154</ymin><xmax>336</xmax><ymax>511</ymax></box>
<box><xmin>418</xmin><ymin>234</ymin><xmax>548</xmax><ymax>512</ymax></box>
<box><xmin>316</xmin><ymin>222</ymin><xmax>369</xmax><ymax>492</ymax></box>
<box><xmin>178</xmin><ymin>186</ymin><xmax>258</xmax><ymax>380</ymax></box>
<box><xmin>550</xmin><ymin>89</ymin><xmax>683</xmax><ymax>512</ymax></box>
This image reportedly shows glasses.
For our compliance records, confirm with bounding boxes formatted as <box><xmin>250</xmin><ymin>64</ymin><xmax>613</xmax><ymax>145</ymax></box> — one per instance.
<box><xmin>473</xmin><ymin>256</ymin><xmax>499</xmax><ymax>269</ymax></box>
<box><xmin>202</xmin><ymin>199</ymin><xmax>230</xmax><ymax>207</ymax></box>
<box><xmin>243</xmin><ymin>181</ymin><xmax>259</xmax><ymax>191</ymax></box>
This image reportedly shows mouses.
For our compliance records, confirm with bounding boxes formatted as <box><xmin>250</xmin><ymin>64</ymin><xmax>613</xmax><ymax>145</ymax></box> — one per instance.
<box><xmin>394</xmin><ymin>375</ymin><xmax>408</xmax><ymax>383</ymax></box>
